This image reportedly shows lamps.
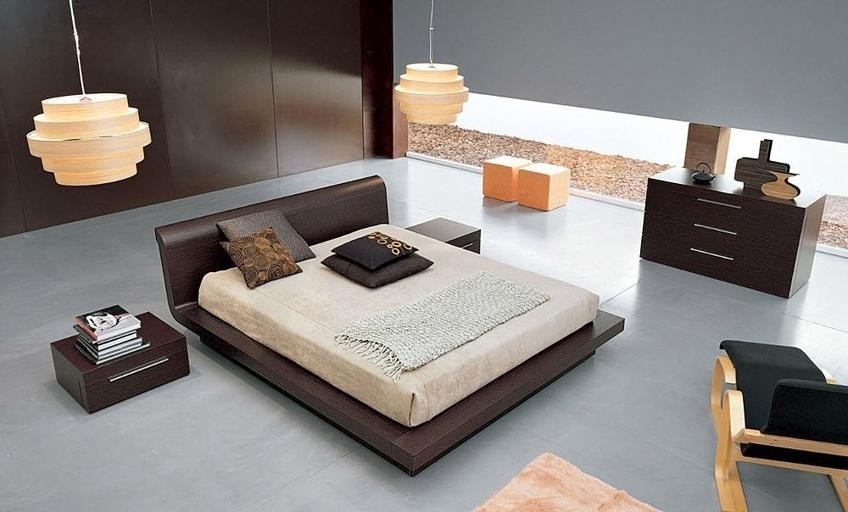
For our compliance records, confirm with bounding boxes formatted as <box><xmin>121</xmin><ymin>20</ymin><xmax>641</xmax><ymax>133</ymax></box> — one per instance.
<box><xmin>391</xmin><ymin>0</ymin><xmax>470</xmax><ymax>126</ymax></box>
<box><xmin>23</xmin><ymin>0</ymin><xmax>154</xmax><ymax>186</ymax></box>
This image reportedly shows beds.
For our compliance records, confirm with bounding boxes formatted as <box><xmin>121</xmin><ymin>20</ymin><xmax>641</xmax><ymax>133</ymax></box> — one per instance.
<box><xmin>152</xmin><ymin>173</ymin><xmax>626</xmax><ymax>478</ymax></box>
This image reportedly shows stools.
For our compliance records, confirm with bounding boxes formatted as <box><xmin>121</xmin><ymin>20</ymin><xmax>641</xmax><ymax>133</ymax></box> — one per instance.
<box><xmin>482</xmin><ymin>155</ymin><xmax>572</xmax><ymax>212</ymax></box>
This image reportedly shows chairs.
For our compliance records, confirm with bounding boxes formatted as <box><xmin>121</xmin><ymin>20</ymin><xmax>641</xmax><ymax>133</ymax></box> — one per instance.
<box><xmin>708</xmin><ymin>337</ymin><xmax>847</xmax><ymax>512</ymax></box>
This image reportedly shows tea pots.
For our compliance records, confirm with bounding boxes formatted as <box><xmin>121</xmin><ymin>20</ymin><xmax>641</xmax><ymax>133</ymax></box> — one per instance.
<box><xmin>691</xmin><ymin>162</ymin><xmax>715</xmax><ymax>185</ymax></box>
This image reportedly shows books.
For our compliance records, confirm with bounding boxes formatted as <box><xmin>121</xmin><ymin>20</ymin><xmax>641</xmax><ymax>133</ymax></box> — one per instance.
<box><xmin>72</xmin><ymin>304</ymin><xmax>152</xmax><ymax>366</ymax></box>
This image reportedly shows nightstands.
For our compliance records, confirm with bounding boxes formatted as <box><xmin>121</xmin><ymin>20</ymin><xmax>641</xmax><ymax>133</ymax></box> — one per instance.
<box><xmin>47</xmin><ymin>311</ymin><xmax>191</xmax><ymax>416</ymax></box>
<box><xmin>405</xmin><ymin>217</ymin><xmax>481</xmax><ymax>257</ymax></box>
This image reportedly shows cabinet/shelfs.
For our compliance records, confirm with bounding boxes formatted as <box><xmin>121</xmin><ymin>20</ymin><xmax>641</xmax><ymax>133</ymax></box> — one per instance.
<box><xmin>638</xmin><ymin>167</ymin><xmax>827</xmax><ymax>299</ymax></box>
<box><xmin>0</xmin><ymin>0</ymin><xmax>388</xmax><ymax>240</ymax></box>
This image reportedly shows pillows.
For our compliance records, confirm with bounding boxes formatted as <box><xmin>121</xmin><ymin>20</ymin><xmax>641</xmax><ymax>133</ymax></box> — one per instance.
<box><xmin>217</xmin><ymin>210</ymin><xmax>436</xmax><ymax>288</ymax></box>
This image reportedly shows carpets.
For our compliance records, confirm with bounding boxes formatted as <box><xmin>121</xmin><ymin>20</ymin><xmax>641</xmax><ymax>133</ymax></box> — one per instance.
<box><xmin>474</xmin><ymin>450</ymin><xmax>665</xmax><ymax>512</ymax></box>
